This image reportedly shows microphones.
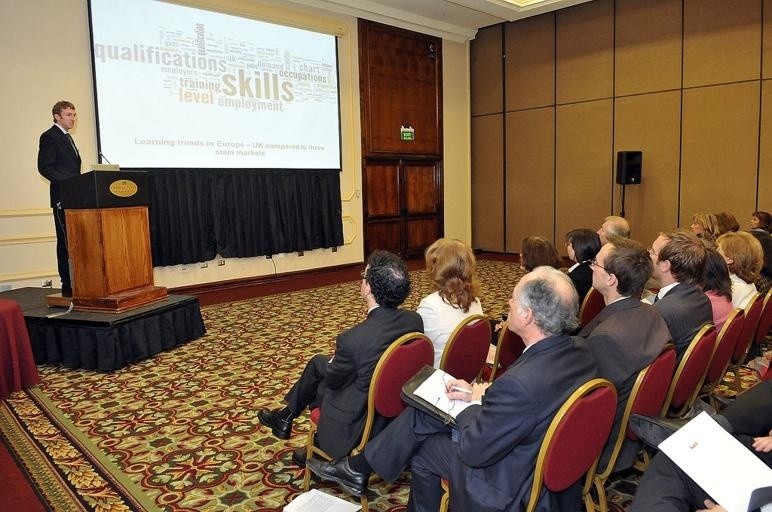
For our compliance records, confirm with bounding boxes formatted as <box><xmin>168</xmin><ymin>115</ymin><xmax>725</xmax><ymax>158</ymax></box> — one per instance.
<box><xmin>99</xmin><ymin>151</ymin><xmax>111</xmax><ymax>165</ymax></box>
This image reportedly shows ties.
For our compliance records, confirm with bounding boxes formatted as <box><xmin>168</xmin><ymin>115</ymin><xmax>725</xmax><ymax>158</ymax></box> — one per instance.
<box><xmin>654</xmin><ymin>294</ymin><xmax>659</xmax><ymax>304</ymax></box>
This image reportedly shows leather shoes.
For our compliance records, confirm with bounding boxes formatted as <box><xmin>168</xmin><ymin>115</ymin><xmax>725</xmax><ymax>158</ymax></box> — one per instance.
<box><xmin>258</xmin><ymin>408</ymin><xmax>292</xmax><ymax>439</ymax></box>
<box><xmin>293</xmin><ymin>447</ymin><xmax>322</xmax><ymax>467</ymax></box>
<box><xmin>629</xmin><ymin>413</ymin><xmax>692</xmax><ymax>449</ymax></box>
<box><xmin>307</xmin><ymin>456</ymin><xmax>369</xmax><ymax>497</ymax></box>
<box><xmin>711</xmin><ymin>394</ymin><xmax>734</xmax><ymax>408</ymax></box>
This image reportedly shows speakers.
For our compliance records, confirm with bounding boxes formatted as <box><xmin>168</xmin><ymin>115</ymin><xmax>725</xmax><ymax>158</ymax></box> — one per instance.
<box><xmin>616</xmin><ymin>151</ymin><xmax>642</xmax><ymax>184</ymax></box>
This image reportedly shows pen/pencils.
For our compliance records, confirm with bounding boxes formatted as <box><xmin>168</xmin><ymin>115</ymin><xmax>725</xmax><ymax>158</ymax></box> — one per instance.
<box><xmin>452</xmin><ymin>386</ymin><xmax>472</xmax><ymax>395</ymax></box>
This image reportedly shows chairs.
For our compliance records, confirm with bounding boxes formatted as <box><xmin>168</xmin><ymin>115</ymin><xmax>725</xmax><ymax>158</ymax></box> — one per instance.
<box><xmin>300</xmin><ymin>330</ymin><xmax>439</xmax><ymax>512</ymax></box>
<box><xmin>436</xmin><ymin>311</ymin><xmax>494</xmax><ymax>386</ymax></box>
<box><xmin>436</xmin><ymin>375</ymin><xmax>621</xmax><ymax>512</ymax></box>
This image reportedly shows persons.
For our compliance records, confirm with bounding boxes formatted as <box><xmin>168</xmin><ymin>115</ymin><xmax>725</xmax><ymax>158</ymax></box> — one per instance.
<box><xmin>257</xmin><ymin>245</ymin><xmax>424</xmax><ymax>464</ymax></box>
<box><xmin>306</xmin><ymin>263</ymin><xmax>600</xmax><ymax>512</ymax></box>
<box><xmin>414</xmin><ymin>235</ymin><xmax>481</xmax><ymax>380</ymax></box>
<box><xmin>37</xmin><ymin>99</ymin><xmax>83</xmax><ymax>296</ymax></box>
<box><xmin>490</xmin><ymin>211</ymin><xmax>772</xmax><ymax>512</ymax></box>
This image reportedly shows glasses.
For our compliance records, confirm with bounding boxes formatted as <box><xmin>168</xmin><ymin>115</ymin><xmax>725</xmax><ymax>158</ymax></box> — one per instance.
<box><xmin>592</xmin><ymin>258</ymin><xmax>610</xmax><ymax>275</ymax></box>
